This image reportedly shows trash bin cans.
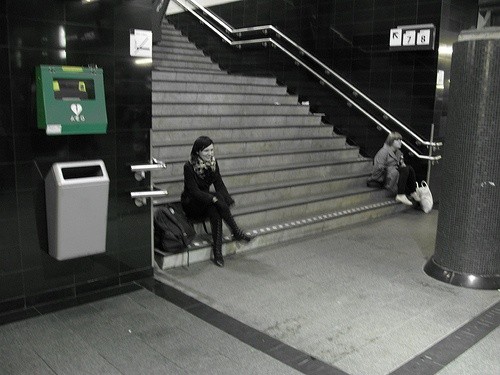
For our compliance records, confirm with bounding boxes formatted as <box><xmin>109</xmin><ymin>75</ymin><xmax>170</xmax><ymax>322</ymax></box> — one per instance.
<box><xmin>44</xmin><ymin>160</ymin><xmax>111</xmax><ymax>261</ymax></box>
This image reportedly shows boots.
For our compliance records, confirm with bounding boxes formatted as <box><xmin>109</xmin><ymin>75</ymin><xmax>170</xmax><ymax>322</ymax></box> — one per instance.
<box><xmin>224</xmin><ymin>214</ymin><xmax>252</xmax><ymax>241</ymax></box>
<box><xmin>212</xmin><ymin>216</ymin><xmax>225</xmax><ymax>267</ymax></box>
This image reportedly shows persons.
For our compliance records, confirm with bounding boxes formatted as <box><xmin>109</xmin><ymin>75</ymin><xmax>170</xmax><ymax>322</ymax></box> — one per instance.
<box><xmin>181</xmin><ymin>136</ymin><xmax>257</xmax><ymax>268</ymax></box>
<box><xmin>374</xmin><ymin>132</ymin><xmax>421</xmax><ymax>205</ymax></box>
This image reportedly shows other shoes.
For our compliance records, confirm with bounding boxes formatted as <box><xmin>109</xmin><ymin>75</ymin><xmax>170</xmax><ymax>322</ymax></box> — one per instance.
<box><xmin>411</xmin><ymin>193</ymin><xmax>421</xmax><ymax>202</ymax></box>
<box><xmin>395</xmin><ymin>194</ymin><xmax>412</xmax><ymax>206</ymax></box>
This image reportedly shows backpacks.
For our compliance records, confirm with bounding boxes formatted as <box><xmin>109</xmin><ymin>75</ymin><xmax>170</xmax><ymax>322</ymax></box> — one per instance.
<box><xmin>154</xmin><ymin>205</ymin><xmax>195</xmax><ymax>253</ymax></box>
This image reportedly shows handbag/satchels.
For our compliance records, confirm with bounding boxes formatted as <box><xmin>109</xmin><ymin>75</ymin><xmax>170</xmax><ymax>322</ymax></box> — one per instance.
<box><xmin>415</xmin><ymin>180</ymin><xmax>433</xmax><ymax>213</ymax></box>
